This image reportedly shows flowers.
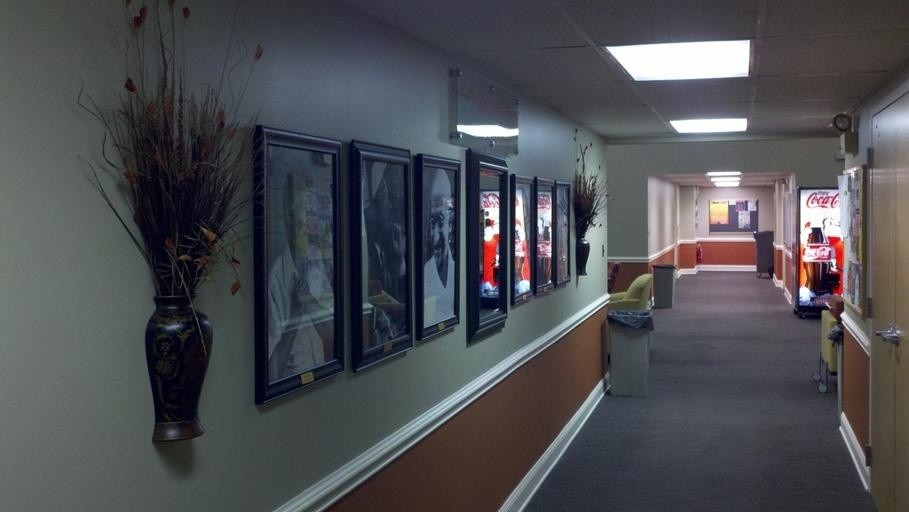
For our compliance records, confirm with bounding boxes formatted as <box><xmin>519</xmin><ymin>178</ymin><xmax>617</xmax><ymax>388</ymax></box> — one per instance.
<box><xmin>66</xmin><ymin>1</ymin><xmax>333</xmax><ymax>358</ymax></box>
<box><xmin>569</xmin><ymin>126</ymin><xmax>623</xmax><ymax>238</ymax></box>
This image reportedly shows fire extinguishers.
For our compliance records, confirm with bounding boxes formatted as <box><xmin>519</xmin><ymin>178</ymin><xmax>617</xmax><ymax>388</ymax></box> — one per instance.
<box><xmin>696</xmin><ymin>243</ymin><xmax>703</xmax><ymax>264</ymax></box>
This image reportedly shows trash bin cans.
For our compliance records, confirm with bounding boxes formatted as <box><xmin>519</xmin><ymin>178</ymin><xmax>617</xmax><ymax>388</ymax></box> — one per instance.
<box><xmin>652</xmin><ymin>263</ymin><xmax>676</xmax><ymax>310</ymax></box>
<box><xmin>608</xmin><ymin>309</ymin><xmax>652</xmax><ymax>398</ymax></box>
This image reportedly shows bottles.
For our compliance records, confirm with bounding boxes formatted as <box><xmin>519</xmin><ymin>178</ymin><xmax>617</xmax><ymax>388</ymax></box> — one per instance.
<box><xmin>803</xmin><ymin>219</ymin><xmax>830</xmax><ymax>297</ymax></box>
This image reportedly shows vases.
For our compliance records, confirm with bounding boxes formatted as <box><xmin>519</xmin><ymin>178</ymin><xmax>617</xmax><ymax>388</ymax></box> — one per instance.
<box><xmin>575</xmin><ymin>236</ymin><xmax>589</xmax><ymax>277</ymax></box>
<box><xmin>143</xmin><ymin>294</ymin><xmax>213</xmax><ymax>445</ymax></box>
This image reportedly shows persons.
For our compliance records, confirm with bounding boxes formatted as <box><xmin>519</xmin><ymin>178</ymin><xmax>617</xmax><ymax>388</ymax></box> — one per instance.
<box><xmin>266</xmin><ymin>170</ymin><xmax>334</xmax><ymax>385</ymax></box>
<box><xmin>422</xmin><ymin>168</ymin><xmax>457</xmax><ymax>330</ymax></box>
<box><xmin>364</xmin><ymin>172</ymin><xmax>405</xmax><ymax>351</ymax></box>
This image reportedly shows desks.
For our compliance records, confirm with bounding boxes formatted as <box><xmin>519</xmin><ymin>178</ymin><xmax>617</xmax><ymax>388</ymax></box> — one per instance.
<box><xmin>811</xmin><ymin>311</ymin><xmax>837</xmax><ymax>395</ymax></box>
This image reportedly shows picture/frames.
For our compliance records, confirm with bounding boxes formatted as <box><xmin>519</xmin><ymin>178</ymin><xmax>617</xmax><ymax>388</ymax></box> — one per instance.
<box><xmin>413</xmin><ymin>150</ymin><xmax>462</xmax><ymax>342</ymax></box>
<box><xmin>555</xmin><ymin>180</ymin><xmax>571</xmax><ymax>286</ymax></box>
<box><xmin>464</xmin><ymin>145</ymin><xmax>510</xmax><ymax>340</ymax></box>
<box><xmin>510</xmin><ymin>172</ymin><xmax>535</xmax><ymax>308</ymax></box>
<box><xmin>535</xmin><ymin>176</ymin><xmax>555</xmax><ymax>296</ymax></box>
<box><xmin>842</xmin><ymin>164</ymin><xmax>868</xmax><ymax>322</ymax></box>
<box><xmin>348</xmin><ymin>136</ymin><xmax>417</xmax><ymax>374</ymax></box>
<box><xmin>255</xmin><ymin>122</ymin><xmax>348</xmax><ymax>404</ymax></box>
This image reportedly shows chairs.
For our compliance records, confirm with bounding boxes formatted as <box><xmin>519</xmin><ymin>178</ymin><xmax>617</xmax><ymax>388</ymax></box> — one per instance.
<box><xmin>608</xmin><ymin>262</ymin><xmax>654</xmax><ymax>364</ymax></box>
